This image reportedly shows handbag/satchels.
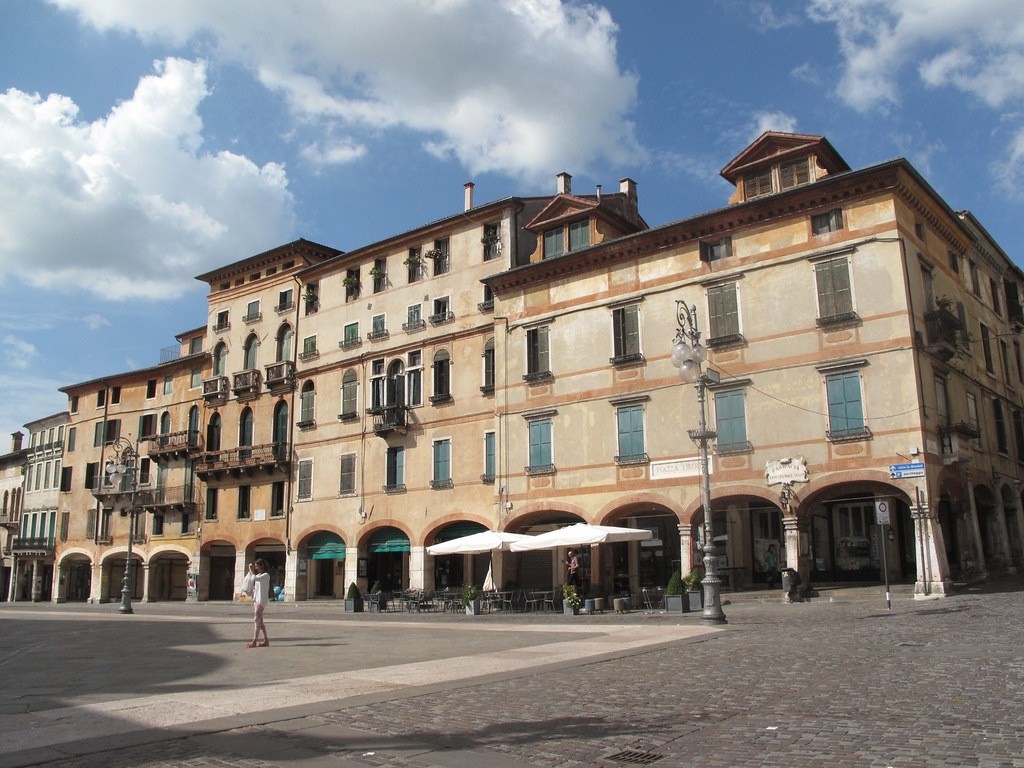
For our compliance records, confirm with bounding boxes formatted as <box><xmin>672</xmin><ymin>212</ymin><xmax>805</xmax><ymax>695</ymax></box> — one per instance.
<box><xmin>241</xmin><ymin>568</ymin><xmax>254</xmax><ymax>594</ymax></box>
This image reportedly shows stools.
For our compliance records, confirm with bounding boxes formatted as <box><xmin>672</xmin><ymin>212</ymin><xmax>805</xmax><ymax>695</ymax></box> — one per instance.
<box><xmin>584</xmin><ymin>593</ymin><xmax>643</xmax><ymax>614</ymax></box>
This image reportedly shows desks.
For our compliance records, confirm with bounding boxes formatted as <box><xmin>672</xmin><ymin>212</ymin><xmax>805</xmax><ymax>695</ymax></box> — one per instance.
<box><xmin>443</xmin><ymin>593</ymin><xmax>462</xmax><ymax>613</ymax></box>
<box><xmin>717</xmin><ymin>566</ymin><xmax>748</xmax><ymax>593</ymax></box>
<box><xmin>365</xmin><ymin>593</ymin><xmax>391</xmax><ymax>613</ymax></box>
<box><xmin>490</xmin><ymin>592</ymin><xmax>517</xmax><ymax>611</ymax></box>
<box><xmin>529</xmin><ymin>591</ymin><xmax>554</xmax><ymax>613</ymax></box>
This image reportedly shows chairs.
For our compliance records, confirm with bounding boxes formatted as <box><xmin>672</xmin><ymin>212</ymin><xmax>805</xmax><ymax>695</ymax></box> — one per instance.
<box><xmin>364</xmin><ymin>583</ymin><xmax>563</xmax><ymax>615</ymax></box>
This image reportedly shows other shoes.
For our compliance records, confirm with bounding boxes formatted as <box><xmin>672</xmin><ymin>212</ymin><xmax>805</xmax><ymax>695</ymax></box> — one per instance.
<box><xmin>245</xmin><ymin>643</ymin><xmax>257</xmax><ymax>648</ymax></box>
<box><xmin>257</xmin><ymin>642</ymin><xmax>269</xmax><ymax>646</ymax></box>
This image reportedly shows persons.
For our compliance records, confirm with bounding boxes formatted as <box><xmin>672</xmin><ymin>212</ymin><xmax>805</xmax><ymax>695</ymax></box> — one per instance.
<box><xmin>245</xmin><ymin>559</ymin><xmax>271</xmax><ymax>648</ymax></box>
<box><xmin>564</xmin><ymin>551</ymin><xmax>578</xmax><ymax>588</ymax></box>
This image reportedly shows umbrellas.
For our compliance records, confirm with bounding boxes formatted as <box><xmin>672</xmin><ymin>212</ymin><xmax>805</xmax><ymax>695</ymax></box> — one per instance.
<box><xmin>425</xmin><ymin>521</ymin><xmax>654</xmax><ymax>592</ymax></box>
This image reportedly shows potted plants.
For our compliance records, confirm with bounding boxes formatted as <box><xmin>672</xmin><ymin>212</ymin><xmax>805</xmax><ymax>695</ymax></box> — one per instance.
<box><xmin>561</xmin><ymin>584</ymin><xmax>583</xmax><ymax>615</ymax></box>
<box><xmin>424</xmin><ymin>248</ymin><xmax>442</xmax><ymax>261</ymax></box>
<box><xmin>665</xmin><ymin>567</ymin><xmax>704</xmax><ymax>614</ymax></box>
<box><xmin>368</xmin><ymin>580</ymin><xmax>385</xmax><ymax>611</ymax></box>
<box><xmin>403</xmin><ymin>255</ymin><xmax>421</xmax><ymax>271</ymax></box>
<box><xmin>276</xmin><ymin>509</ymin><xmax>283</xmax><ymax>516</ymax></box>
<box><xmin>344</xmin><ymin>582</ymin><xmax>363</xmax><ymax>613</ymax></box>
<box><xmin>302</xmin><ymin>289</ymin><xmax>318</xmax><ymax>303</ymax></box>
<box><xmin>933</xmin><ymin>324</ymin><xmax>947</xmax><ymax>343</ymax></box>
<box><xmin>462</xmin><ymin>584</ymin><xmax>480</xmax><ymax>615</ymax></box>
<box><xmin>481</xmin><ymin>229</ymin><xmax>496</xmax><ymax>244</ymax></box>
<box><xmin>342</xmin><ymin>274</ymin><xmax>359</xmax><ymax>287</ymax></box>
<box><xmin>369</xmin><ymin>267</ymin><xmax>383</xmax><ymax>279</ymax></box>
